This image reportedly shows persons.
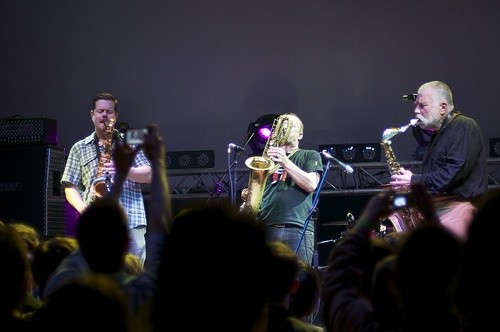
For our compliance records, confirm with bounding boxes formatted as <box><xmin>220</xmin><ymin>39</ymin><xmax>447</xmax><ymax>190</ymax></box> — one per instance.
<box><xmin>240</xmin><ymin>113</ymin><xmax>324</xmax><ymax>267</ymax></box>
<box><xmin>0</xmin><ymin>124</ymin><xmax>500</xmax><ymax>332</ymax></box>
<box><xmin>389</xmin><ymin>80</ymin><xmax>487</xmax><ymax>203</ymax></box>
<box><xmin>61</xmin><ymin>92</ymin><xmax>151</xmax><ymax>264</ymax></box>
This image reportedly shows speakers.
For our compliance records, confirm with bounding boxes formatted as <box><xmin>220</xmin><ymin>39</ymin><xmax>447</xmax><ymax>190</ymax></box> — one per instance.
<box><xmin>0</xmin><ymin>143</ymin><xmax>66</xmax><ymax>238</ymax></box>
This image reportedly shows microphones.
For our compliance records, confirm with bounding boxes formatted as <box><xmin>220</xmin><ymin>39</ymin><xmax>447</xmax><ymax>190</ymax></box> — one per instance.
<box><xmin>229</xmin><ymin>142</ymin><xmax>245</xmax><ymax>152</ymax></box>
<box><xmin>402</xmin><ymin>94</ymin><xmax>417</xmax><ymax>101</ymax></box>
<box><xmin>322</xmin><ymin>151</ymin><xmax>353</xmax><ymax>175</ymax></box>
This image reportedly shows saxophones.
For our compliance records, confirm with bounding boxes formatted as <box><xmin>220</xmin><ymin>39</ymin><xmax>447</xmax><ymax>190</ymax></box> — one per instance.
<box><xmin>242</xmin><ymin>113</ymin><xmax>294</xmax><ymax>217</ymax></box>
<box><xmin>90</xmin><ymin>118</ymin><xmax>115</xmax><ymax>198</ymax></box>
<box><xmin>379</xmin><ymin>116</ymin><xmax>421</xmax><ymax>233</ymax></box>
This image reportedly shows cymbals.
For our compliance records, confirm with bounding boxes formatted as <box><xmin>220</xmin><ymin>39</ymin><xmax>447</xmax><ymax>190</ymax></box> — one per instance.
<box><xmin>316</xmin><ymin>238</ymin><xmax>341</xmax><ymax>245</ymax></box>
<box><xmin>321</xmin><ymin>220</ymin><xmax>359</xmax><ymax>226</ymax></box>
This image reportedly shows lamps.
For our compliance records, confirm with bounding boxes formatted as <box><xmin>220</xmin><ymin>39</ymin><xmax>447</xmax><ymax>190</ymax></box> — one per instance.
<box><xmin>490</xmin><ymin>137</ymin><xmax>500</xmax><ymax>158</ymax></box>
<box><xmin>163</xmin><ymin>149</ymin><xmax>214</xmax><ymax>169</ymax></box>
<box><xmin>246</xmin><ymin>115</ymin><xmax>279</xmax><ymax>151</ymax></box>
<box><xmin>318</xmin><ymin>142</ymin><xmax>381</xmax><ymax>163</ymax></box>
